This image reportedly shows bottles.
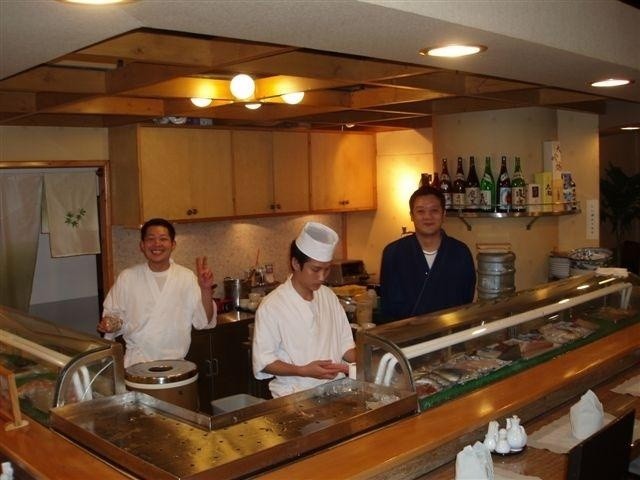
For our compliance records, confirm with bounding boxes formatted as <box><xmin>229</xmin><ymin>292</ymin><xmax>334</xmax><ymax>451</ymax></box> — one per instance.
<box><xmin>451</xmin><ymin>157</ymin><xmax>466</xmax><ymax>211</ymax></box>
<box><xmin>496</xmin><ymin>156</ymin><xmax>511</xmax><ymax>212</ymax></box>
<box><xmin>511</xmin><ymin>157</ymin><xmax>527</xmax><ymax>212</ymax></box>
<box><xmin>480</xmin><ymin>156</ymin><xmax>496</xmax><ymax>212</ymax></box>
<box><xmin>465</xmin><ymin>156</ymin><xmax>480</xmax><ymax>212</ymax></box>
<box><xmin>438</xmin><ymin>157</ymin><xmax>451</xmax><ymax>211</ymax></box>
<box><xmin>431</xmin><ymin>172</ymin><xmax>439</xmax><ymax>188</ymax></box>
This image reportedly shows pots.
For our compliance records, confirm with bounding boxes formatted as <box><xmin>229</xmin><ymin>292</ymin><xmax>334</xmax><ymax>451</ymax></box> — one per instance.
<box><xmin>223</xmin><ymin>277</ymin><xmax>253</xmax><ymax>312</ymax></box>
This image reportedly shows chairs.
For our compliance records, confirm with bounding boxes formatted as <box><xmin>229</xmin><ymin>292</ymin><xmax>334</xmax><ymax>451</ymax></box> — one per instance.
<box><xmin>566</xmin><ymin>409</ymin><xmax>636</xmax><ymax>479</ymax></box>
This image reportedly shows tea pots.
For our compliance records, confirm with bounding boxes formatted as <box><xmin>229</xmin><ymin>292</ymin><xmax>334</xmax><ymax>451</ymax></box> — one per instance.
<box><xmin>506</xmin><ymin>415</ymin><xmax>527</xmax><ymax>451</ymax></box>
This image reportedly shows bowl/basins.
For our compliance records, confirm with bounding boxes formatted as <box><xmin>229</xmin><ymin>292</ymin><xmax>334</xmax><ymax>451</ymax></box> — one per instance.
<box><xmin>567</xmin><ymin>247</ymin><xmax>616</xmax><ymax>269</ymax></box>
<box><xmin>249</xmin><ymin>301</ymin><xmax>258</xmax><ymax>312</ymax></box>
<box><xmin>257</xmin><ymin>297</ymin><xmax>263</xmax><ymax>305</ymax></box>
<box><xmin>249</xmin><ymin>292</ymin><xmax>259</xmax><ymax>301</ymax></box>
<box><xmin>240</xmin><ymin>299</ymin><xmax>250</xmax><ymax>309</ymax></box>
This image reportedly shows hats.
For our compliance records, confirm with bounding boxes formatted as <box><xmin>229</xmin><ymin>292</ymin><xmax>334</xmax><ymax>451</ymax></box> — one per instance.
<box><xmin>296</xmin><ymin>221</ymin><xmax>340</xmax><ymax>263</ymax></box>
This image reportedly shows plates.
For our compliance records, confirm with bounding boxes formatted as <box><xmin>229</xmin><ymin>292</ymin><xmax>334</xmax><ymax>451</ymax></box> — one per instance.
<box><xmin>549</xmin><ymin>262</ymin><xmax>570</xmax><ymax>279</ymax></box>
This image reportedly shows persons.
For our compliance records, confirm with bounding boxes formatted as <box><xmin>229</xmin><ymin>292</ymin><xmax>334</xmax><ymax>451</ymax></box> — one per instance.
<box><xmin>380</xmin><ymin>186</ymin><xmax>476</xmax><ymax>375</ymax></box>
<box><xmin>252</xmin><ymin>223</ymin><xmax>357</xmax><ymax>399</ymax></box>
<box><xmin>97</xmin><ymin>218</ymin><xmax>217</xmax><ymax>369</ymax></box>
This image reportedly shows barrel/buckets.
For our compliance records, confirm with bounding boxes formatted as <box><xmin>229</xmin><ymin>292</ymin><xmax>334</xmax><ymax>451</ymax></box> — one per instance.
<box><xmin>477</xmin><ymin>252</ymin><xmax>516</xmax><ymax>299</ymax></box>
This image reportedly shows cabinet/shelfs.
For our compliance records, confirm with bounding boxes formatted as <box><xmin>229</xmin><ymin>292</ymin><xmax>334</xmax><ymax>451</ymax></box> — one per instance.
<box><xmin>188</xmin><ymin>321</ymin><xmax>249</xmax><ymax>410</ymax></box>
<box><xmin>109</xmin><ymin>123</ymin><xmax>236</xmax><ymax>228</ymax></box>
<box><xmin>308</xmin><ymin>130</ymin><xmax>377</xmax><ymax>213</ymax></box>
<box><xmin>231</xmin><ymin>126</ymin><xmax>310</xmax><ymax>218</ymax></box>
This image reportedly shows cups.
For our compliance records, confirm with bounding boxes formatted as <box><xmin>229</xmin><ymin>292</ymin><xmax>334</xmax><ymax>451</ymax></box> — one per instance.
<box><xmin>104</xmin><ymin>313</ymin><xmax>120</xmax><ymax>333</ymax></box>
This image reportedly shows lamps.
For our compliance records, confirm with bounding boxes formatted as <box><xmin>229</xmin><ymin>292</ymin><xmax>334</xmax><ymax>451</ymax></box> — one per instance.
<box><xmin>230</xmin><ymin>73</ymin><xmax>255</xmax><ymax>100</ymax></box>
<box><xmin>191</xmin><ymin>98</ymin><xmax>212</xmax><ymax>107</ymax></box>
<box><xmin>282</xmin><ymin>92</ymin><xmax>304</xmax><ymax>104</ymax></box>
<box><xmin>244</xmin><ymin>104</ymin><xmax>261</xmax><ymax>110</ymax></box>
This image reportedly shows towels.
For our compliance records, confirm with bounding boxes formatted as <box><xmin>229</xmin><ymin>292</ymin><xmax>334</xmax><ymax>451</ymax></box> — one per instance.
<box><xmin>526</xmin><ymin>411</ymin><xmax>640</xmax><ymax>453</ymax></box>
<box><xmin>610</xmin><ymin>372</ymin><xmax>639</xmax><ymax>399</ymax></box>
